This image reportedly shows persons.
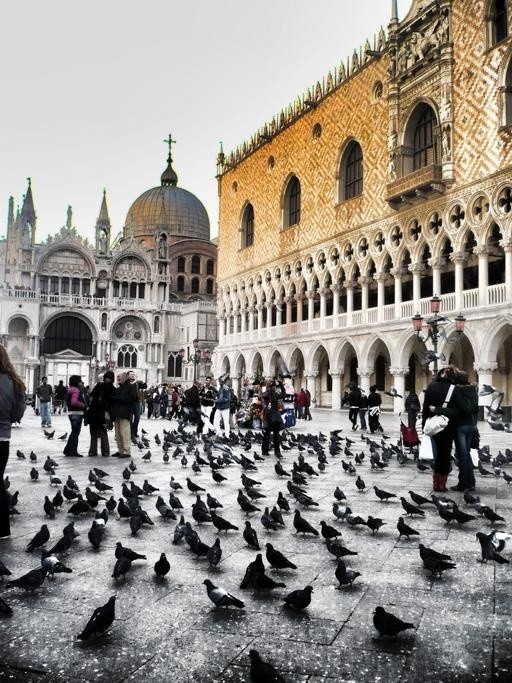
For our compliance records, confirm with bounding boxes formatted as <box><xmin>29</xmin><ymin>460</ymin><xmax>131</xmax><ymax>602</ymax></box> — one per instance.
<box><xmin>421</xmin><ymin>366</ymin><xmax>480</xmax><ymax>491</ymax></box>
<box><xmin>341</xmin><ymin>381</ymin><xmax>383</xmax><ymax>434</ymax></box>
<box><xmin>99</xmin><ymin>234</ymin><xmax>107</xmax><ymax>253</ymax></box>
<box><xmin>52</xmin><ymin>370</ymin><xmax>312</xmax><ymax>459</ymax></box>
<box><xmin>404</xmin><ymin>390</ymin><xmax>420</xmax><ymax>428</ymax></box>
<box><xmin>37</xmin><ymin>377</ymin><xmax>53</xmax><ymax>428</ymax></box>
<box><xmin>429</xmin><ymin>371</ymin><xmax>478</xmax><ymax>492</ymax></box>
<box><xmin>0</xmin><ymin>344</ymin><xmax>26</xmax><ymax>540</ymax></box>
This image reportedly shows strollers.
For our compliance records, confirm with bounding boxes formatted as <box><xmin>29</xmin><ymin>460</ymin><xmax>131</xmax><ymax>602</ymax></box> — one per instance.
<box><xmin>397</xmin><ymin>412</ymin><xmax>422</xmax><ymax>466</ymax></box>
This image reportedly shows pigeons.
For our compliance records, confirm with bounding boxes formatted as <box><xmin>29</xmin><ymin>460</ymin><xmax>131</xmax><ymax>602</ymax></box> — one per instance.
<box><xmin>0</xmin><ymin>418</ymin><xmax>512</xmax><ymax>595</ymax></box>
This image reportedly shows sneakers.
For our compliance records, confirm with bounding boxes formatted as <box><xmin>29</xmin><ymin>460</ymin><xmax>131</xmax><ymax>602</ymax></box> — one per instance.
<box><xmin>295</xmin><ymin>415</ymin><xmax>312</xmax><ymax>421</ymax></box>
<box><xmin>11</xmin><ymin>422</ymin><xmax>20</xmax><ymax>428</ymax></box>
<box><xmin>262</xmin><ymin>452</ymin><xmax>270</xmax><ymax>456</ymax></box>
<box><xmin>143</xmin><ymin>416</ymin><xmax>179</xmax><ymax>421</ymax></box>
<box><xmin>42</xmin><ymin>424</ymin><xmax>52</xmax><ymax>427</ymax></box>
<box><xmin>88</xmin><ymin>453</ymin><xmax>109</xmax><ymax>457</ymax></box>
<box><xmin>351</xmin><ymin>424</ymin><xmax>384</xmax><ymax>434</ymax></box>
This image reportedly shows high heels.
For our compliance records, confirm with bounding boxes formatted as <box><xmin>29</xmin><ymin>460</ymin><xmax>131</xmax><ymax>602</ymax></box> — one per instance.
<box><xmin>450</xmin><ymin>480</ymin><xmax>476</xmax><ymax>492</ymax></box>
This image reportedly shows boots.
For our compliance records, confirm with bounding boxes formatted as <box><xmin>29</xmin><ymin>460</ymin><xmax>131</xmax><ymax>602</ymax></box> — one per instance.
<box><xmin>433</xmin><ymin>474</ymin><xmax>448</xmax><ymax>492</ymax></box>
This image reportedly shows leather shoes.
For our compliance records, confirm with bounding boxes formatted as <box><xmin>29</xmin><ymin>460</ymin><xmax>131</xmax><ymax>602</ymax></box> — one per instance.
<box><xmin>63</xmin><ymin>451</ymin><xmax>83</xmax><ymax>457</ymax></box>
<box><xmin>111</xmin><ymin>452</ymin><xmax>131</xmax><ymax>458</ymax></box>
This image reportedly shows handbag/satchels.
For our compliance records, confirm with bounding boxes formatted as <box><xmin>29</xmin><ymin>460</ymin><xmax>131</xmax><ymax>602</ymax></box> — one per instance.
<box><xmin>422</xmin><ymin>415</ymin><xmax>449</xmax><ymax>435</ymax></box>
<box><xmin>471</xmin><ymin>428</ymin><xmax>480</xmax><ymax>450</ymax></box>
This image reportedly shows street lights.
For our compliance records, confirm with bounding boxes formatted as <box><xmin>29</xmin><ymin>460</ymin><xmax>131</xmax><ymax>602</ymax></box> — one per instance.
<box><xmin>410</xmin><ymin>293</ymin><xmax>468</xmax><ymax>380</ymax></box>
<box><xmin>179</xmin><ymin>338</ymin><xmax>211</xmax><ymax>386</ymax></box>
<box><xmin>22</xmin><ymin>355</ymin><xmax>37</xmax><ymax>397</ymax></box>
<box><xmin>93</xmin><ymin>352</ymin><xmax>117</xmax><ymax>372</ymax></box>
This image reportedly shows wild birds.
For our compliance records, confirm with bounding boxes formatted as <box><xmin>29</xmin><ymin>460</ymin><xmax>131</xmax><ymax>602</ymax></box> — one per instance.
<box><xmin>0</xmin><ymin>596</ymin><xmax>14</xmax><ymax>617</ymax></box>
<box><xmin>247</xmin><ymin>649</ymin><xmax>285</xmax><ymax>682</ymax></box>
<box><xmin>76</xmin><ymin>596</ymin><xmax>116</xmax><ymax>643</ymax></box>
<box><xmin>371</xmin><ymin>606</ymin><xmax>415</xmax><ymax>641</ymax></box>
<box><xmin>279</xmin><ymin>585</ymin><xmax>315</xmax><ymax>609</ymax></box>
<box><xmin>202</xmin><ymin>579</ymin><xmax>246</xmax><ymax>612</ymax></box>
